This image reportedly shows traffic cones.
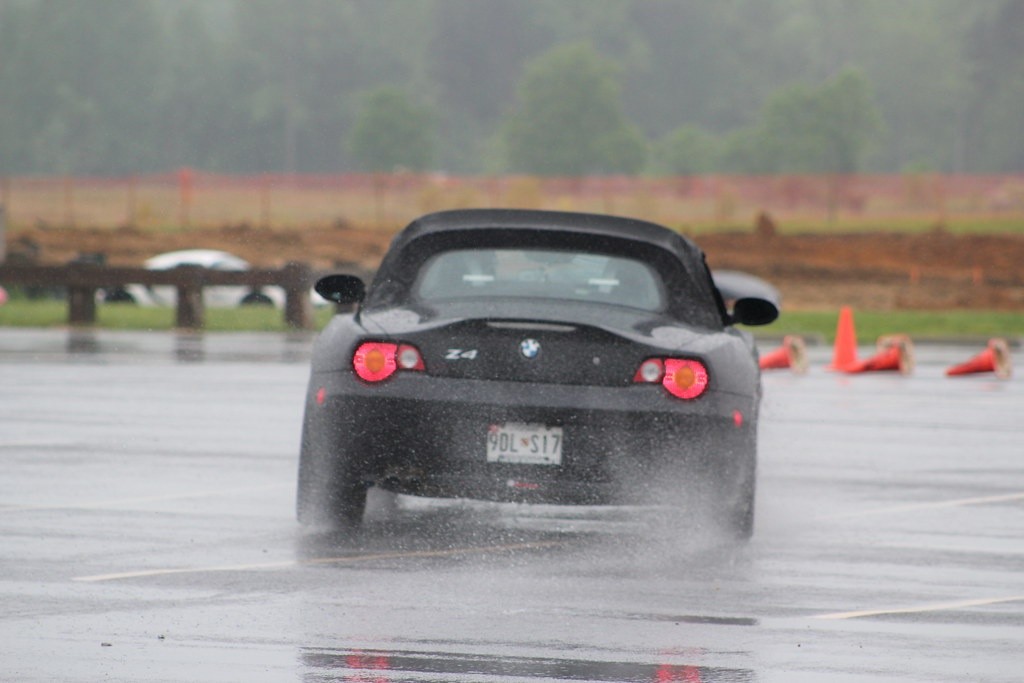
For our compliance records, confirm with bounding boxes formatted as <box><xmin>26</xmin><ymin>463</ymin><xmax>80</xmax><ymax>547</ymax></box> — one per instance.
<box><xmin>825</xmin><ymin>305</ymin><xmax>864</xmax><ymax>373</ymax></box>
<box><xmin>946</xmin><ymin>338</ymin><xmax>1014</xmax><ymax>379</ymax></box>
<box><xmin>758</xmin><ymin>336</ymin><xmax>806</xmax><ymax>375</ymax></box>
<box><xmin>861</xmin><ymin>333</ymin><xmax>920</xmax><ymax>375</ymax></box>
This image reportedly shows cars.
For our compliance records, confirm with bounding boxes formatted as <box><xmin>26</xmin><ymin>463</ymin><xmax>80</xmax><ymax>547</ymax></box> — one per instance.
<box><xmin>91</xmin><ymin>246</ymin><xmax>328</xmax><ymax>306</ymax></box>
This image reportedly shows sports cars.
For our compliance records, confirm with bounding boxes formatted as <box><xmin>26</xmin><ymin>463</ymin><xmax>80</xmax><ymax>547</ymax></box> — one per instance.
<box><xmin>298</xmin><ymin>208</ymin><xmax>778</xmax><ymax>551</ymax></box>
